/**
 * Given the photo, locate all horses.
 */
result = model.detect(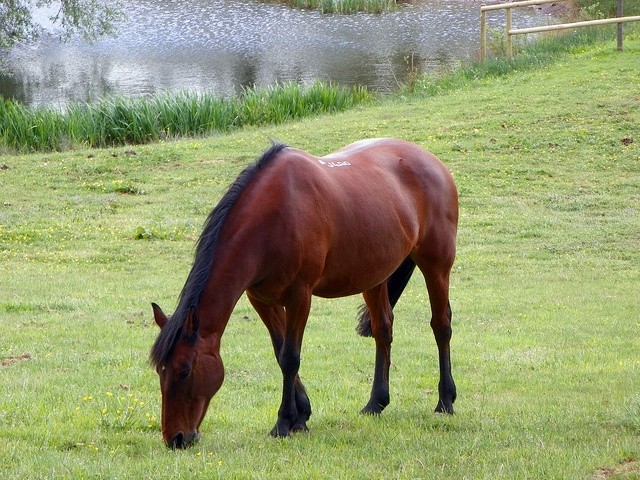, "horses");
[147,137,460,452]
